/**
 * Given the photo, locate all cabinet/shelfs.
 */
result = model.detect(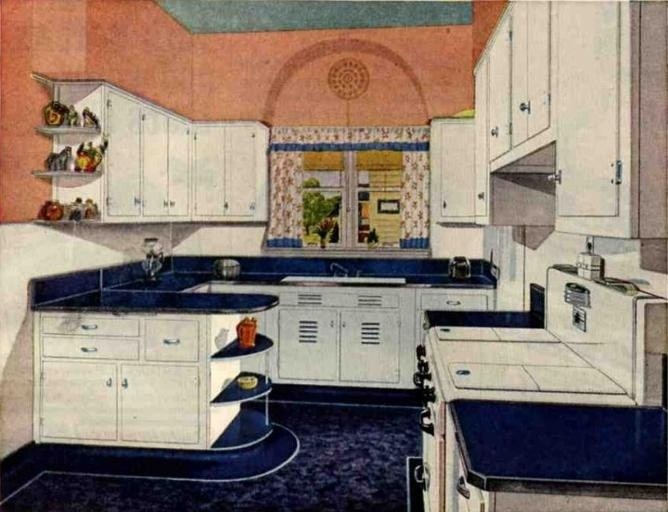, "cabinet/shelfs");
[421,290,490,342]
[210,301,280,447]
[107,91,142,216]
[279,291,400,386]
[39,313,205,451]
[143,108,190,216]
[473,57,488,216]
[414,343,483,512]
[195,125,255,218]
[441,123,477,218]
[511,2,550,151]
[34,76,104,224]
[485,15,512,162]
[554,0,622,219]
